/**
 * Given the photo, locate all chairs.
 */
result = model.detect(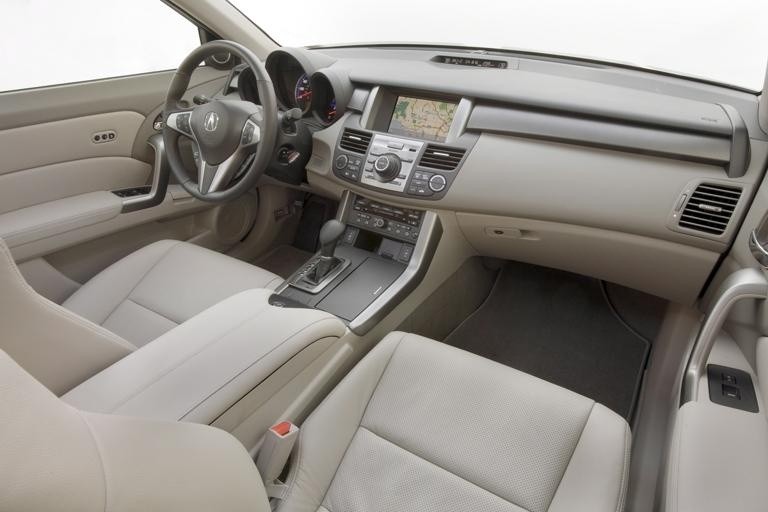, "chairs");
[1,328,634,508]
[2,234,288,394]
